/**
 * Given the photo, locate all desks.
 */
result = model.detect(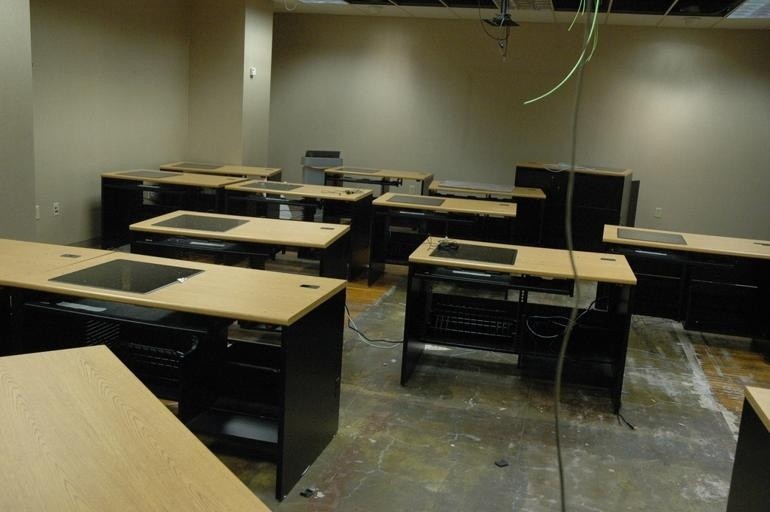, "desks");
[322,160,640,271]
[400,229,637,419]
[99,168,372,281]
[2,233,347,502]
[601,222,769,358]
[0,346,273,512]
